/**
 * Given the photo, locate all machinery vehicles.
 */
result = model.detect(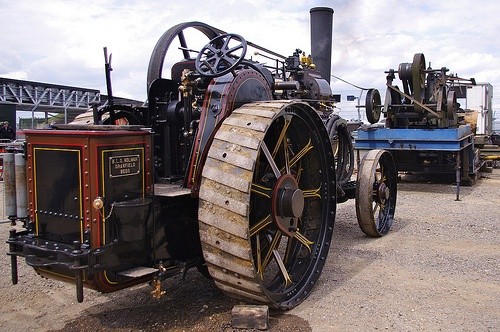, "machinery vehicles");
[1,5,401,332]
[348,49,499,202]
